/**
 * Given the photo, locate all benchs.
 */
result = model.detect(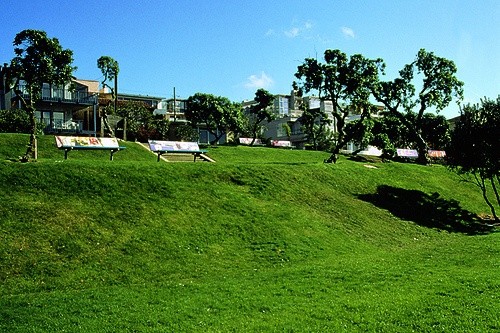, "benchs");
[270,140,292,149]
[427,150,448,163]
[147,139,209,163]
[395,147,420,163]
[54,135,126,161]
[239,137,268,147]
